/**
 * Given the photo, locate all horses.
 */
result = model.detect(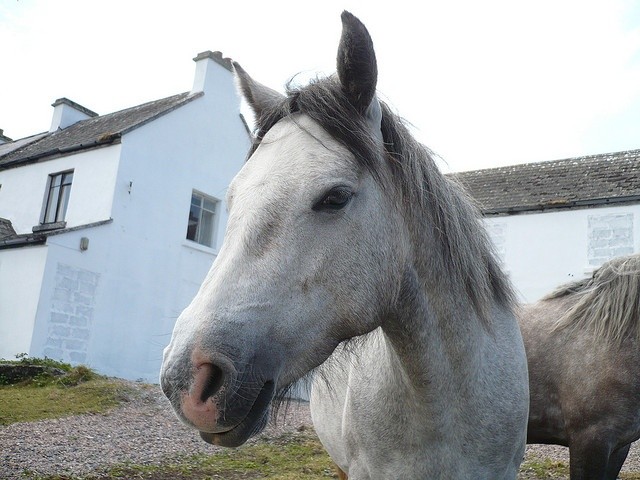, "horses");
[159,9,530,479]
[508,252,640,480]
